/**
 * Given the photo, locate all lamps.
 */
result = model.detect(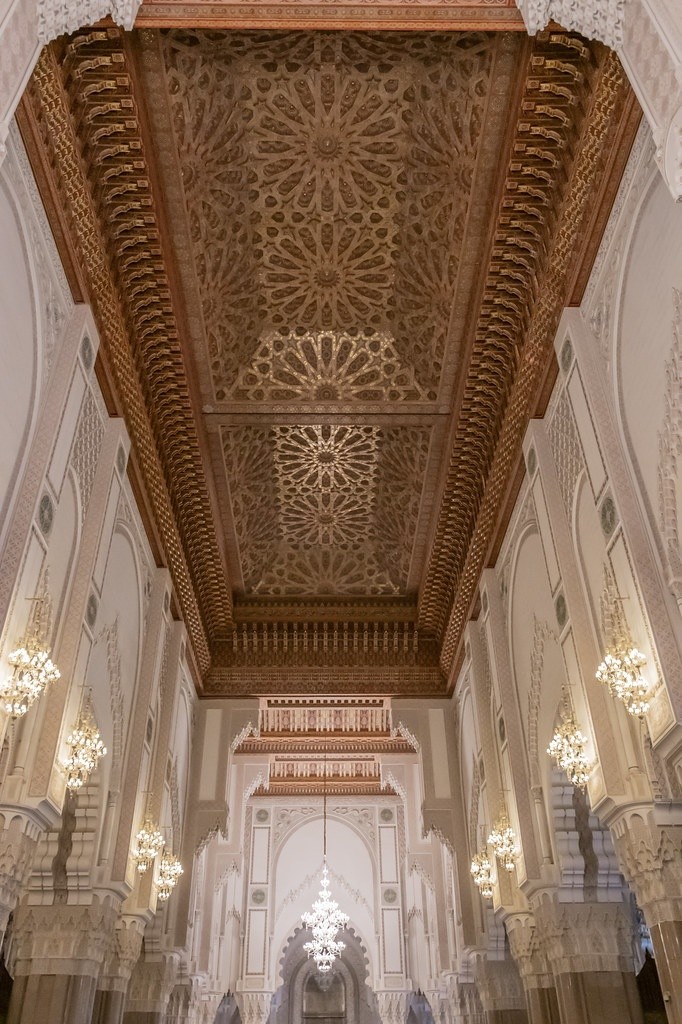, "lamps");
[153,823,185,901]
[297,753,352,973]
[0,596,65,727]
[544,681,593,797]
[468,823,498,901]
[485,789,522,878]
[57,682,108,801]
[126,787,168,876]
[591,561,652,724]
